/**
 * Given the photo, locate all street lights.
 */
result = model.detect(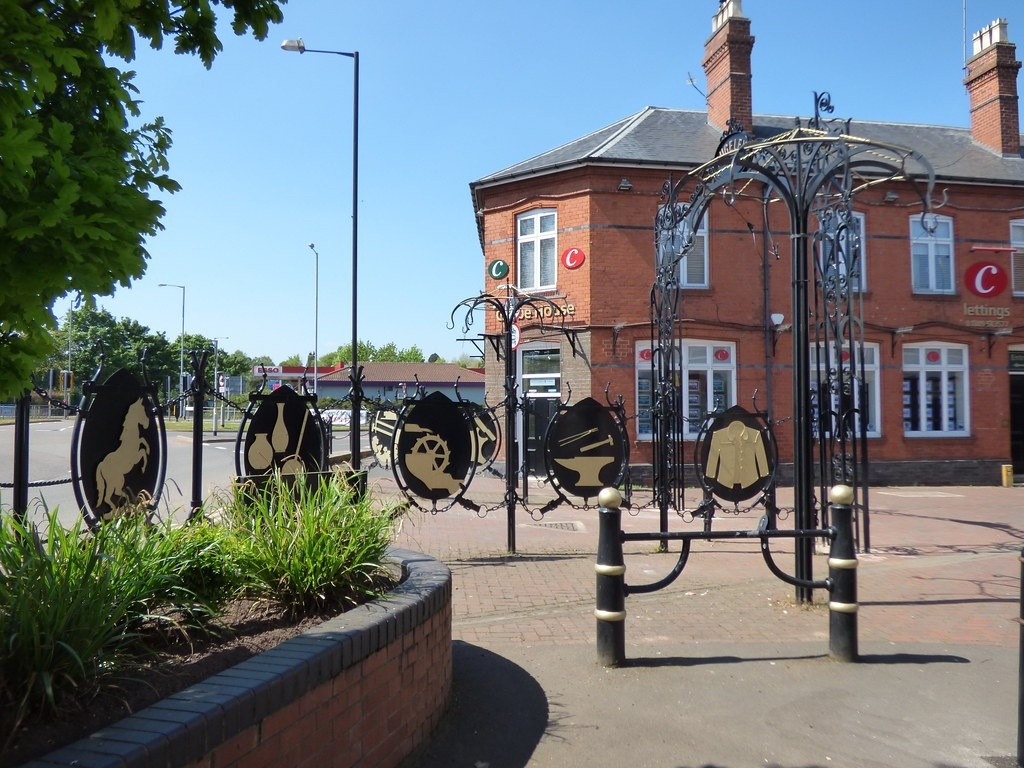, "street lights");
[69,299,77,372]
[158,284,185,416]
[307,244,319,398]
[280,38,360,472]
